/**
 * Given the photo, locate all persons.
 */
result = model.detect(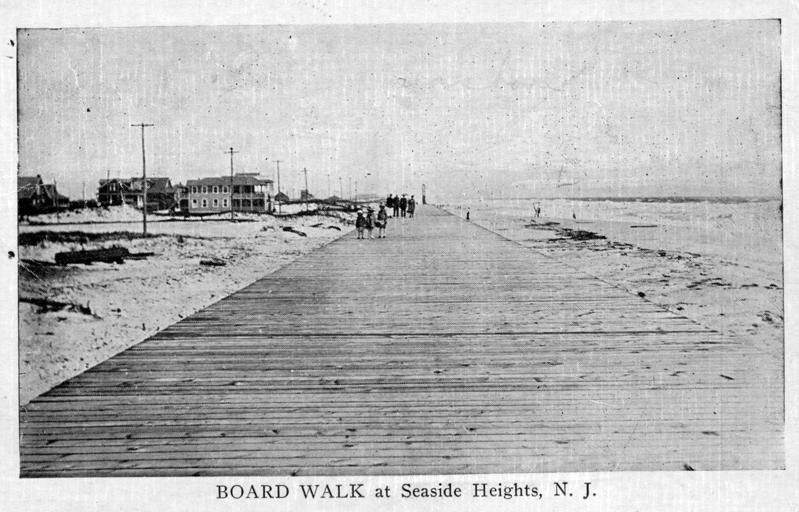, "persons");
[355,210,366,239]
[407,195,416,219]
[375,201,387,239]
[393,194,400,217]
[399,194,407,218]
[385,193,393,217]
[366,205,377,240]
